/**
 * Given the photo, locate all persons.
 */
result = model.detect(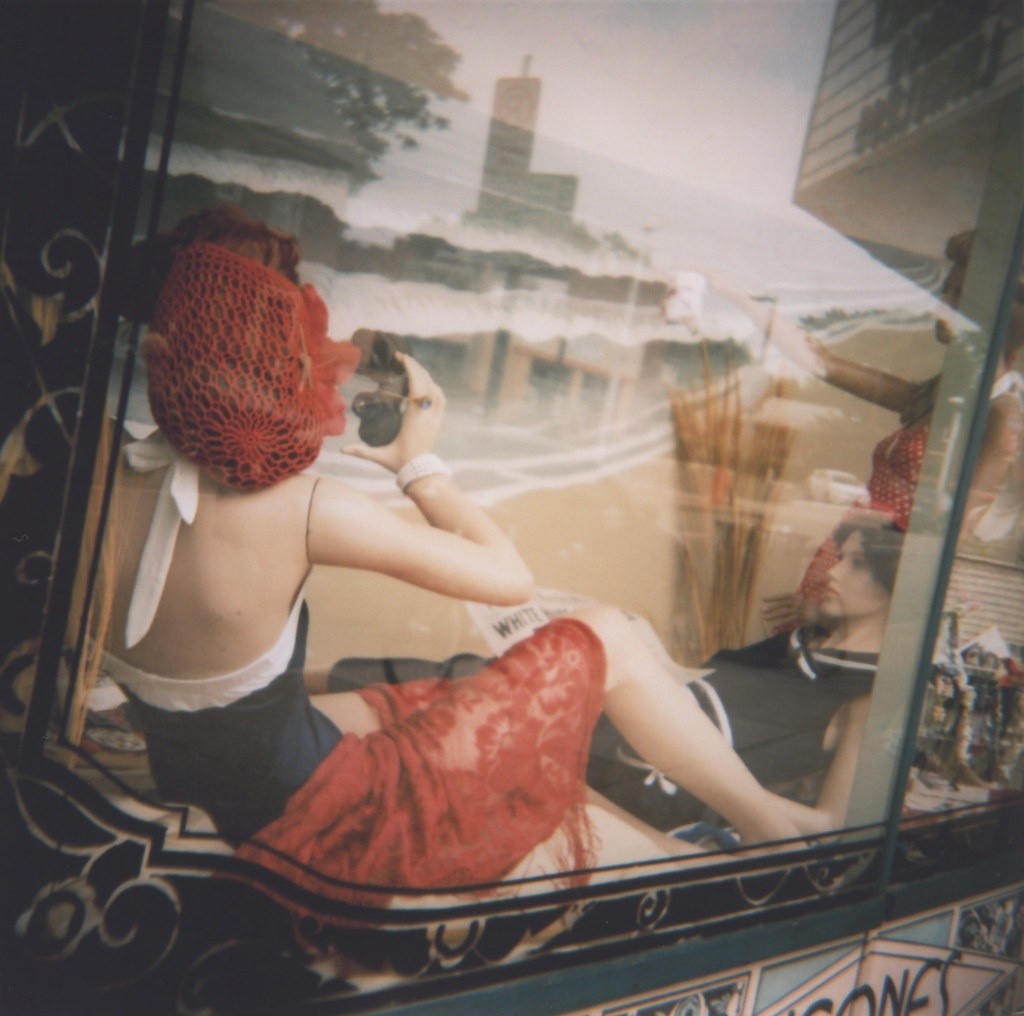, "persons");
[695,230,1022,634]
[85,246,844,886]
[394,450,448,491]
[294,513,906,831]
